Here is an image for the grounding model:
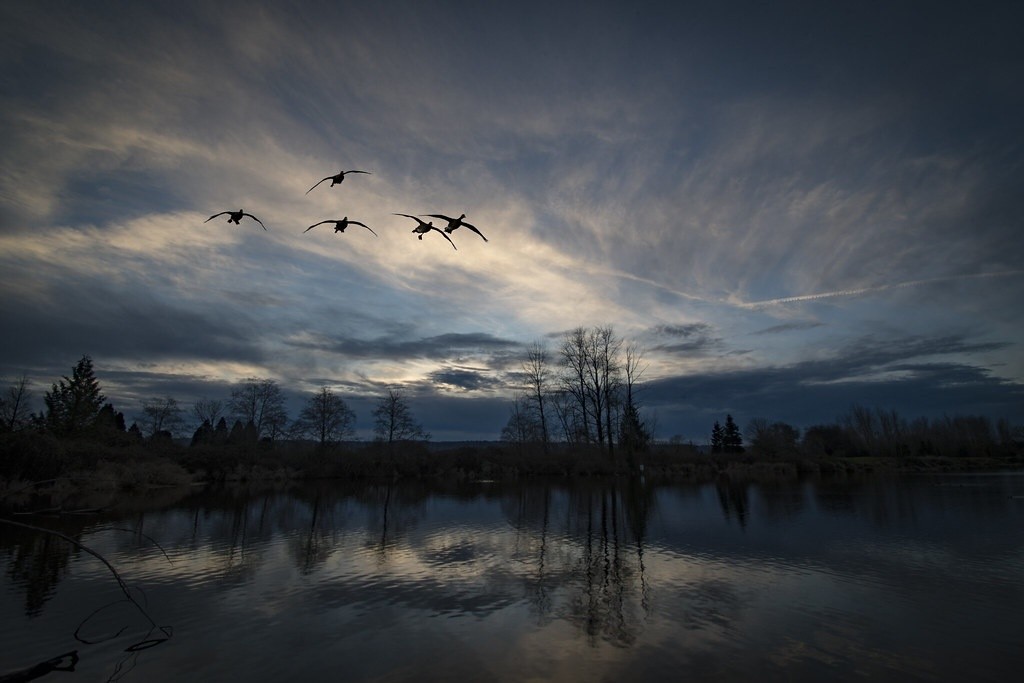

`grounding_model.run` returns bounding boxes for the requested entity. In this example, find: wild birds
[305,170,371,196]
[204,208,267,232]
[303,216,378,236]
[417,213,489,243]
[392,212,457,251]
